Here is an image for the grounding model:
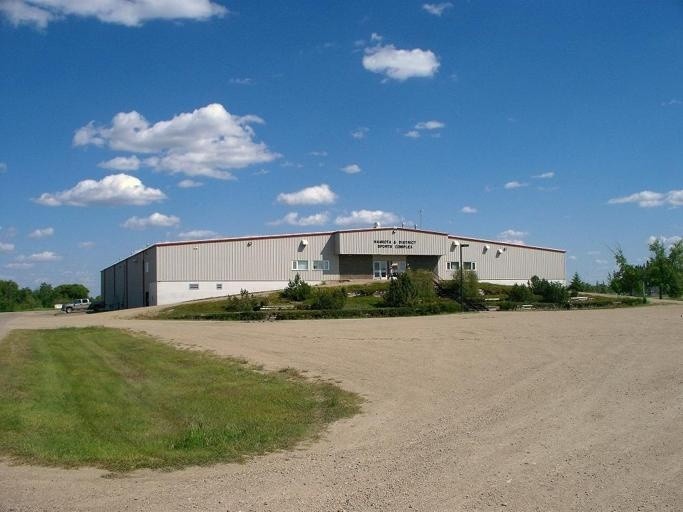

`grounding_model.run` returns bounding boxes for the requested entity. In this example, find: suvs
[61,299,93,313]
